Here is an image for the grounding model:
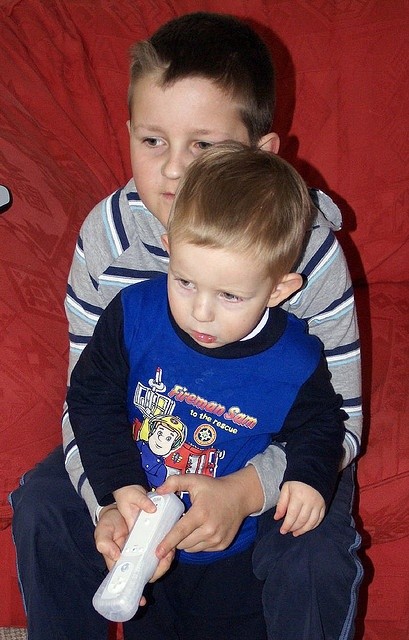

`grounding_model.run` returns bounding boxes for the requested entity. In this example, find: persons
[9,11,365,639]
[66,139,349,639]
